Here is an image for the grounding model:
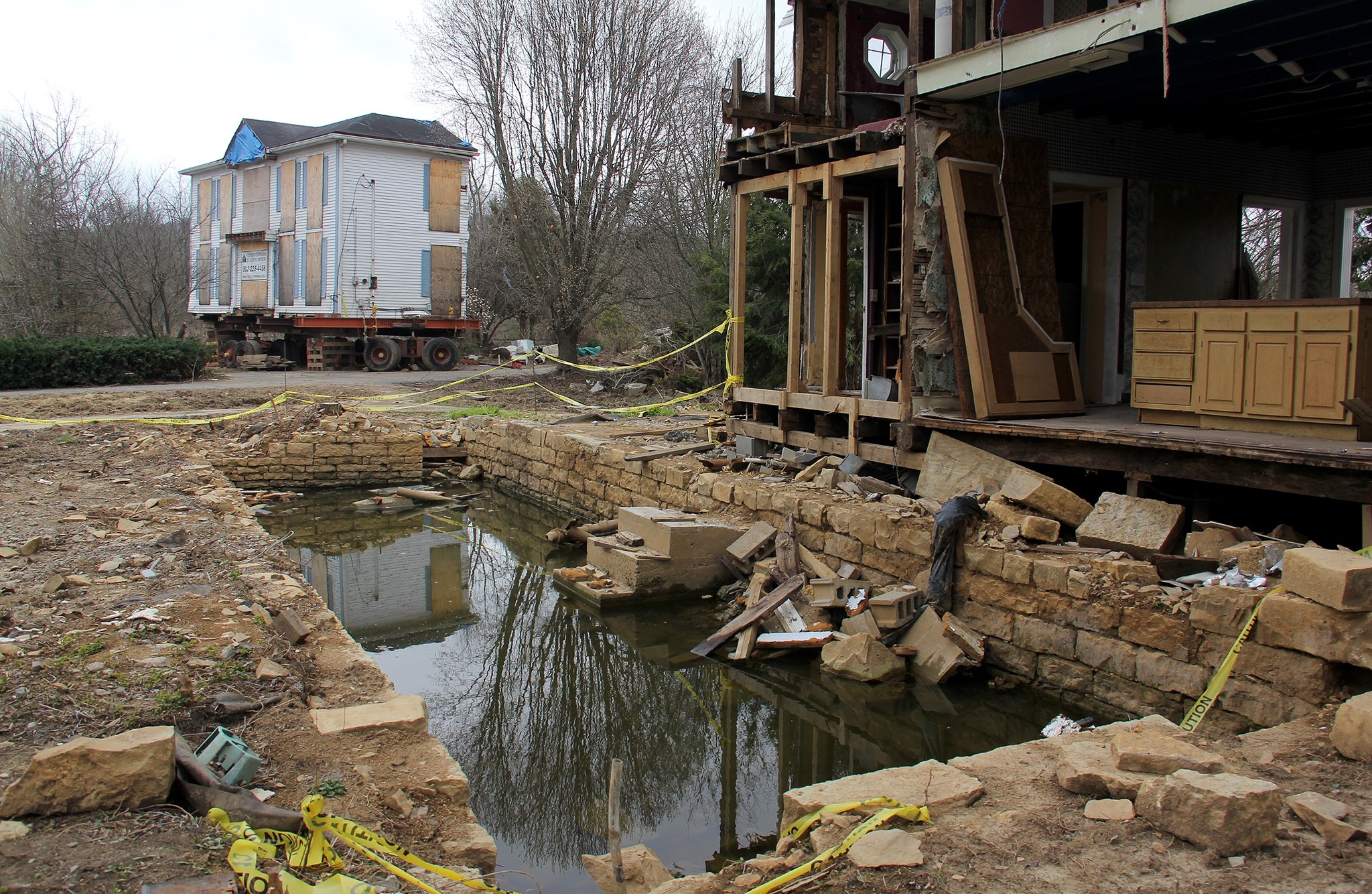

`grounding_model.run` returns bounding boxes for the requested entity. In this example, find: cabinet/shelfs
[1131,304,1372,442]
[881,204,901,377]
